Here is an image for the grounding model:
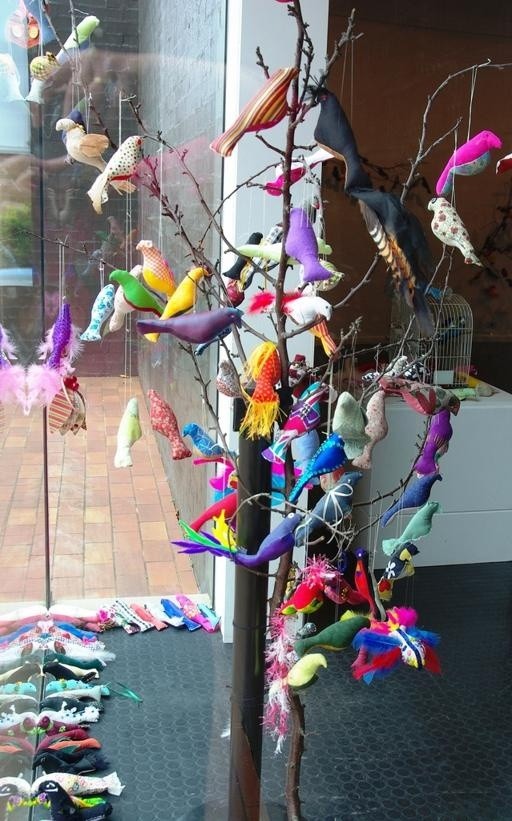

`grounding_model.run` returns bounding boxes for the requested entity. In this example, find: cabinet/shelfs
[347,375,512,572]
